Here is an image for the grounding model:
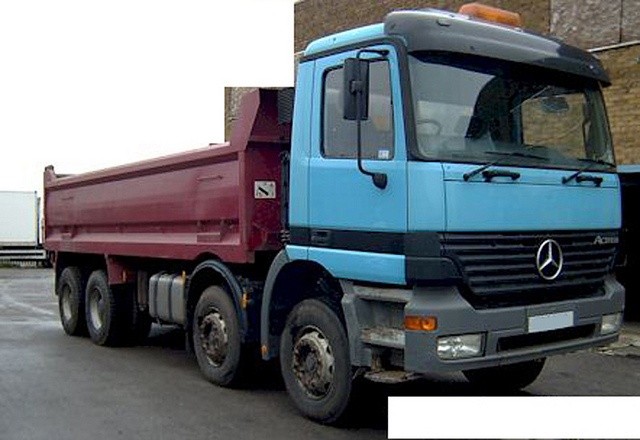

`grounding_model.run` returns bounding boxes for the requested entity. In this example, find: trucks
[0,190,52,268]
[43,3,624,424]
